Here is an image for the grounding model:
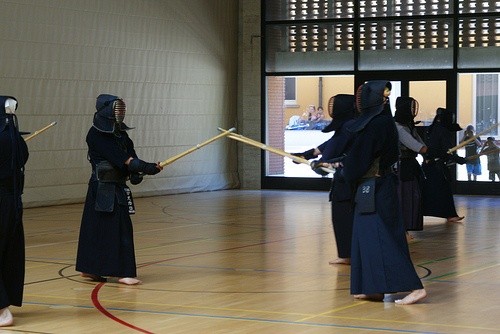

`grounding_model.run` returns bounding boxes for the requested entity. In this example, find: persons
[290,80,500,305]
[0,96,29,327]
[75,94,163,285]
